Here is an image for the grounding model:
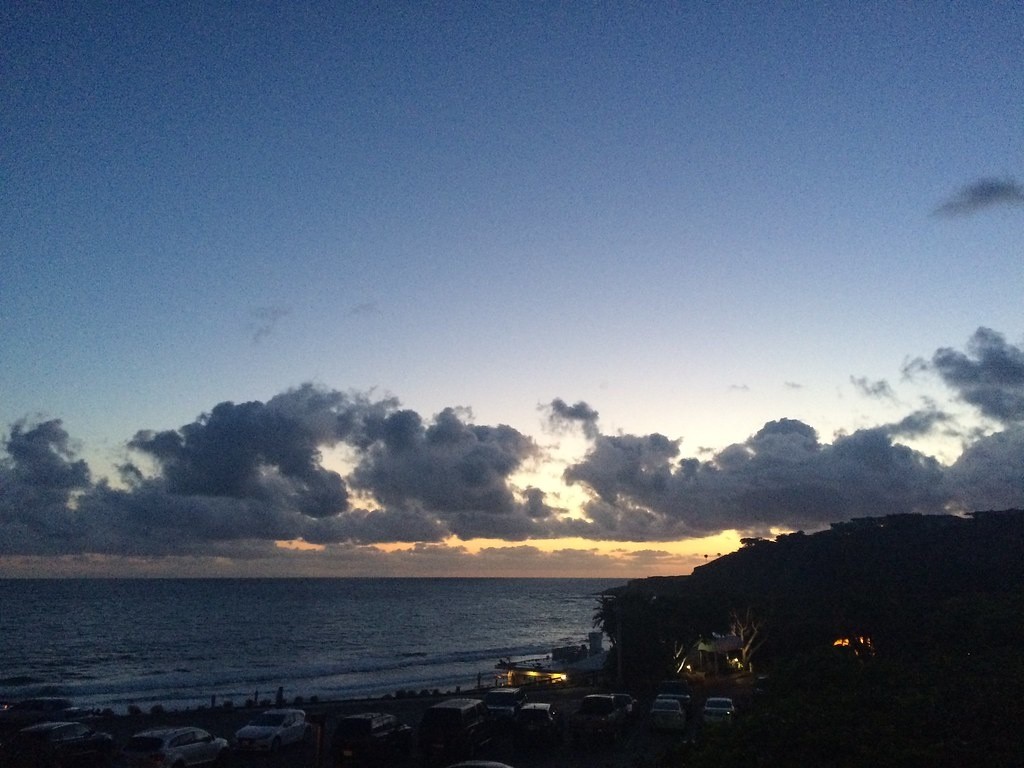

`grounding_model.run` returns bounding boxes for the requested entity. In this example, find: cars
[0,721,114,764]
[329,713,413,764]
[416,698,497,755]
[703,697,735,722]
[487,688,528,718]
[117,727,230,768]
[0,698,69,727]
[611,693,637,713]
[649,700,687,733]
[233,709,313,753]
[657,680,693,708]
[517,703,559,729]
[569,695,621,729]
